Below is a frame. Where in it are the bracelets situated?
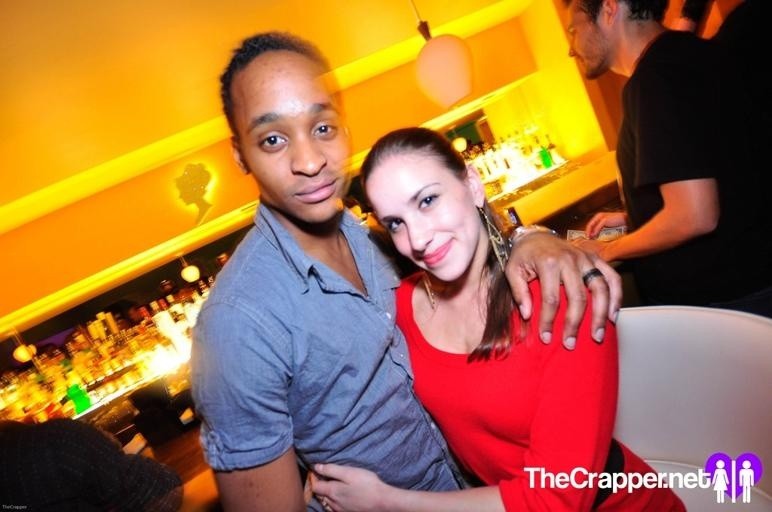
[507,225,556,249]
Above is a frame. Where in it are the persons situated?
[189,32,624,511]
[305,126,686,511]
[567,0,725,317]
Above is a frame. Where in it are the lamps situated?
[410,0,474,110]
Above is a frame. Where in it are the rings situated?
[581,267,605,288]
[318,496,325,504]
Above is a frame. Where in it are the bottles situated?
[0,251,228,422]
[468,129,563,175]
